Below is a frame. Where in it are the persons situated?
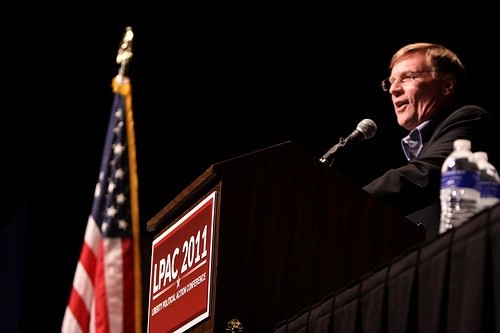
[362,42,500,241]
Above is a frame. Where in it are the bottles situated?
[474,152,500,213]
[440,139,480,234]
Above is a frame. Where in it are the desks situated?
[270,200,500,333]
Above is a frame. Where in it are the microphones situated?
[320,119,378,164]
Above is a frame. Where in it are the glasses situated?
[381,70,431,91]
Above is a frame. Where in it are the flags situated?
[61,75,143,333]
